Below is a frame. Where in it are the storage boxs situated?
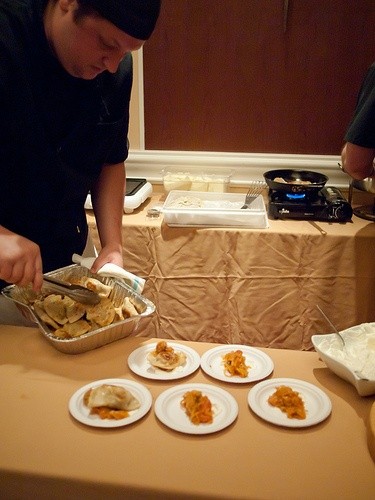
[160,190,270,229]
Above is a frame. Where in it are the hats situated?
[87,0,161,41]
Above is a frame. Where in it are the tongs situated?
[29,272,99,305]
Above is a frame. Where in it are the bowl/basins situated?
[310,322,375,396]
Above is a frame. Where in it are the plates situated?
[127,343,201,380]
[154,383,239,434]
[248,378,332,427]
[69,378,153,428]
[200,345,275,383]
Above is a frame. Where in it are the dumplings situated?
[88,385,140,410]
[32,275,141,340]
[147,351,187,370]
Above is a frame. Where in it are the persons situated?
[342,60,375,179]
[0,0,161,330]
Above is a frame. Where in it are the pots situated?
[262,169,329,194]
[338,162,375,195]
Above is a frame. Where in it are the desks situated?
[82,184,375,352]
[0,323,375,500]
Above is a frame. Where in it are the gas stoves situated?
[267,186,354,223]
